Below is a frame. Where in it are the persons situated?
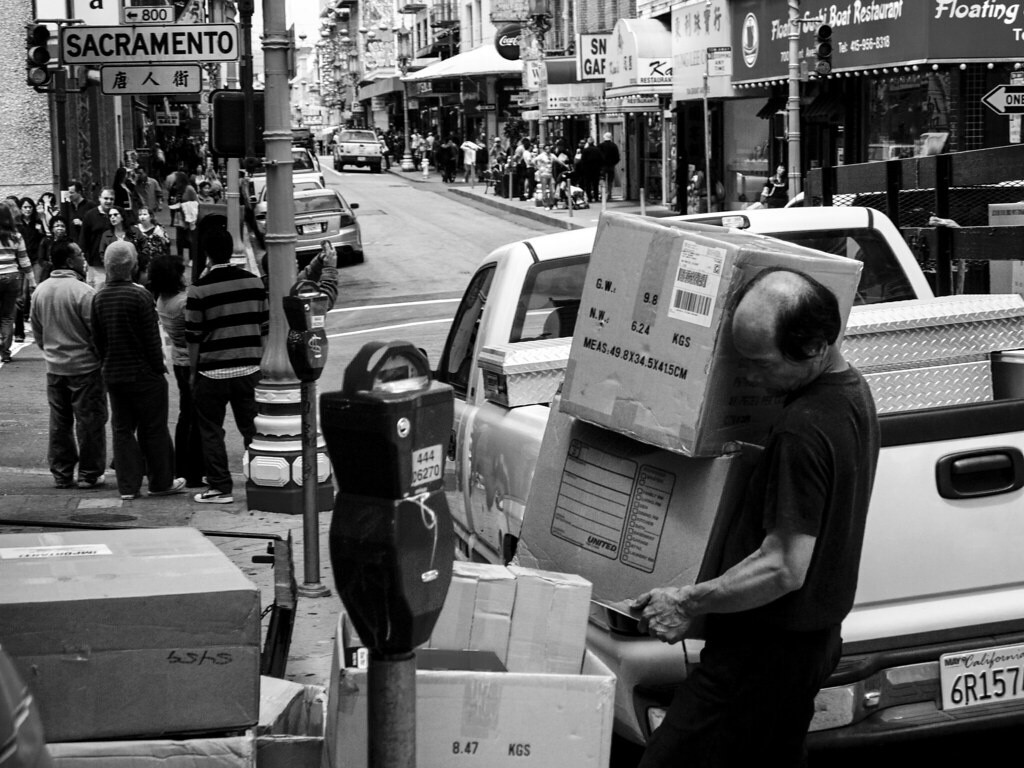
[760,163,788,207]
[627,268,881,768]
[89,241,187,500]
[149,255,208,487]
[30,237,119,490]
[262,243,338,312]
[376,123,488,183]
[505,132,621,210]
[185,230,269,503]
[0,128,222,362]
[490,137,505,159]
[318,131,339,155]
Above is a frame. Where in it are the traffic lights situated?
[812,25,832,75]
[25,24,51,86]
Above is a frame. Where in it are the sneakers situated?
[122,491,141,499]
[193,487,234,503]
[78,475,105,488]
[148,477,186,495]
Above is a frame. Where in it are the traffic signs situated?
[982,86,1024,113]
[473,103,496,113]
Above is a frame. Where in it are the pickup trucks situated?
[332,128,382,172]
[415,205,1024,746]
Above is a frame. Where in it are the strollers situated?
[556,170,589,210]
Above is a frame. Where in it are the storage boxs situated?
[843,295,1024,415]
[477,337,574,407]
[990,203,1023,296]
[510,384,761,625]
[320,560,617,768]
[0,526,328,768]
[559,209,867,457]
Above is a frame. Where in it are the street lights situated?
[527,0,554,206]
[396,18,416,171]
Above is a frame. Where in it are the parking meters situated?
[318,335,455,759]
[283,276,332,600]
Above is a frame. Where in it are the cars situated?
[238,128,364,267]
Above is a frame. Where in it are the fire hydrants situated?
[420,158,429,178]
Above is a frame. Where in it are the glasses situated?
[109,213,120,217]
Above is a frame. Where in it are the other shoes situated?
[3,357,11,362]
[14,334,24,342]
